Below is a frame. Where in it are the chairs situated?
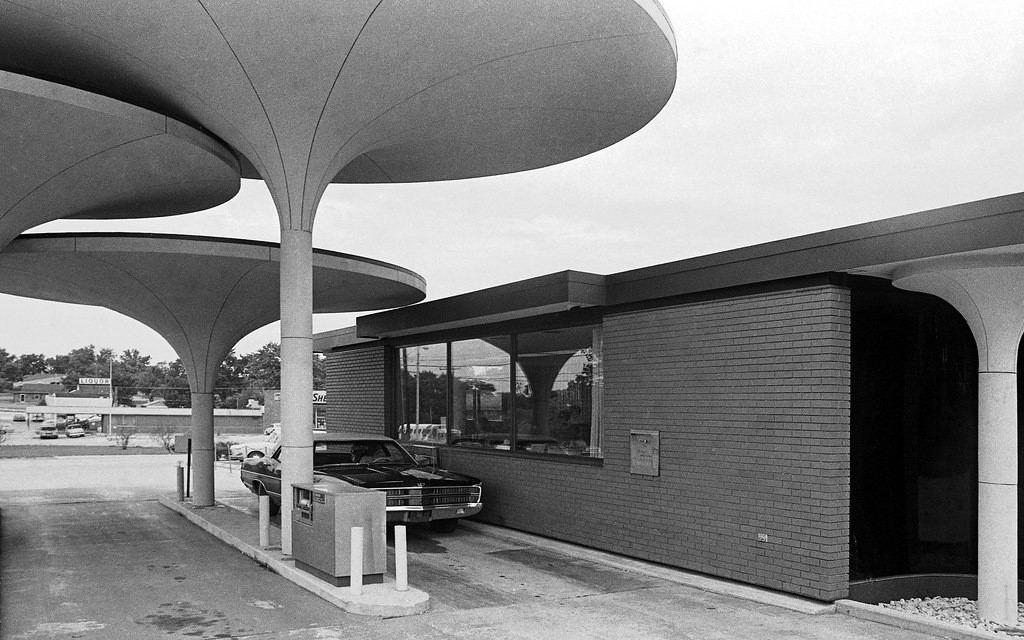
[351,449,376,463]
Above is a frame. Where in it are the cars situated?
[241,432,483,533]
[398,424,591,453]
[40,426,59,439]
[232,431,328,463]
[14,413,26,421]
[65,423,86,438]
[32,414,44,422]
[264,423,281,435]
[42,416,101,433]
